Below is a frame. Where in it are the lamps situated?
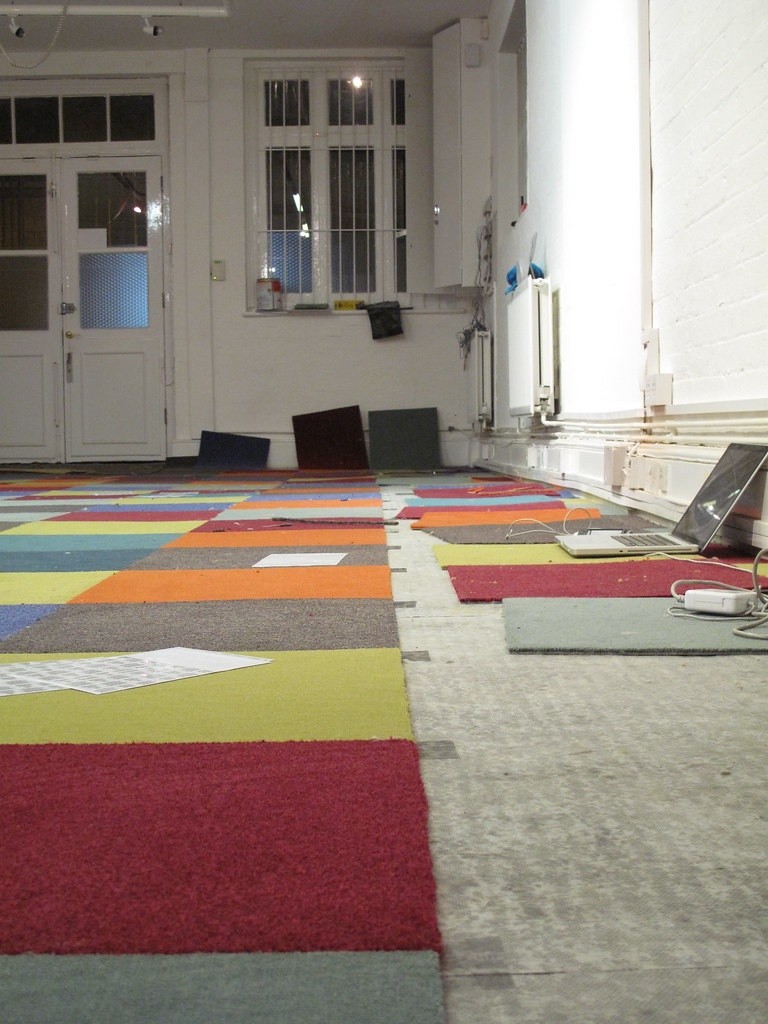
[6,14,26,38]
[142,14,163,36]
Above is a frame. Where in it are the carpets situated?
[0,470,447,1023]
[397,476,768,654]
[290,405,369,471]
[367,407,443,473]
[190,430,271,471]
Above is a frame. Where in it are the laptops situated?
[554,442,768,558]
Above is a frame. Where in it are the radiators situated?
[507,275,555,418]
[467,328,492,424]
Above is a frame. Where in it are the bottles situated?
[257,278,282,311]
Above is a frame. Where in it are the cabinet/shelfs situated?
[404,18,489,298]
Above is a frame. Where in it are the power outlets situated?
[645,371,674,405]
